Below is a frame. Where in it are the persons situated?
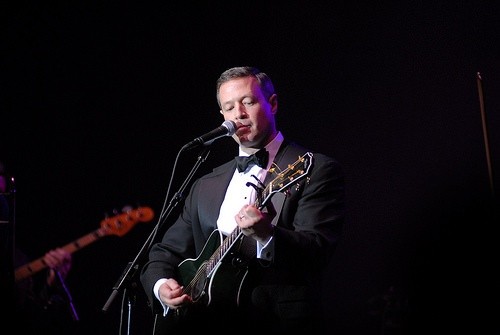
[140,65,342,335]
[0,176,70,335]
[398,186,500,335]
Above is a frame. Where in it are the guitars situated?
[13,205,155,281]
[153,151,315,335]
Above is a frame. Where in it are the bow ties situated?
[235,149,269,173]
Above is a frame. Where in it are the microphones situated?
[182,119,237,150]
